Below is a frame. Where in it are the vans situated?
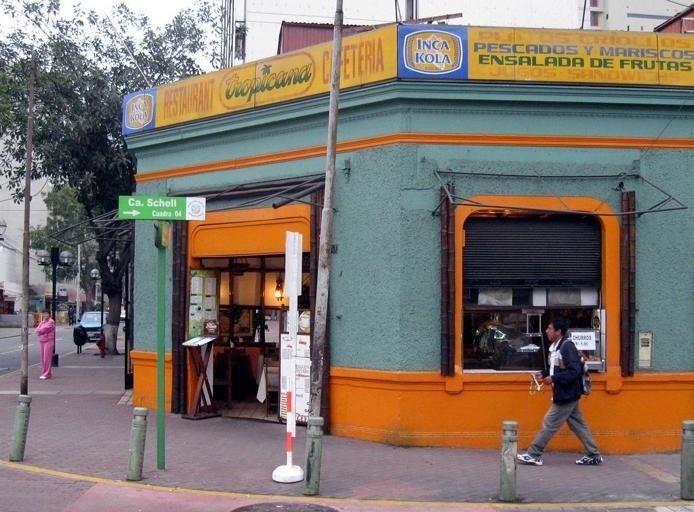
[77,312,108,343]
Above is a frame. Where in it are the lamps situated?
[274,272,284,300]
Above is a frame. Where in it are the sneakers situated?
[575,456,604,467]
[40,376,50,380]
[517,452,544,467]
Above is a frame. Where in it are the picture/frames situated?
[219,308,255,337]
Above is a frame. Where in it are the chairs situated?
[256,353,280,418]
[211,347,232,408]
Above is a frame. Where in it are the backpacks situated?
[559,339,592,396]
[474,325,498,356]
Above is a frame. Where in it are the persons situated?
[35,309,54,380]
[515,316,605,465]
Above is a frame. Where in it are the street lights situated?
[0,216,8,240]
[36,247,73,353]
[90,268,104,358]
